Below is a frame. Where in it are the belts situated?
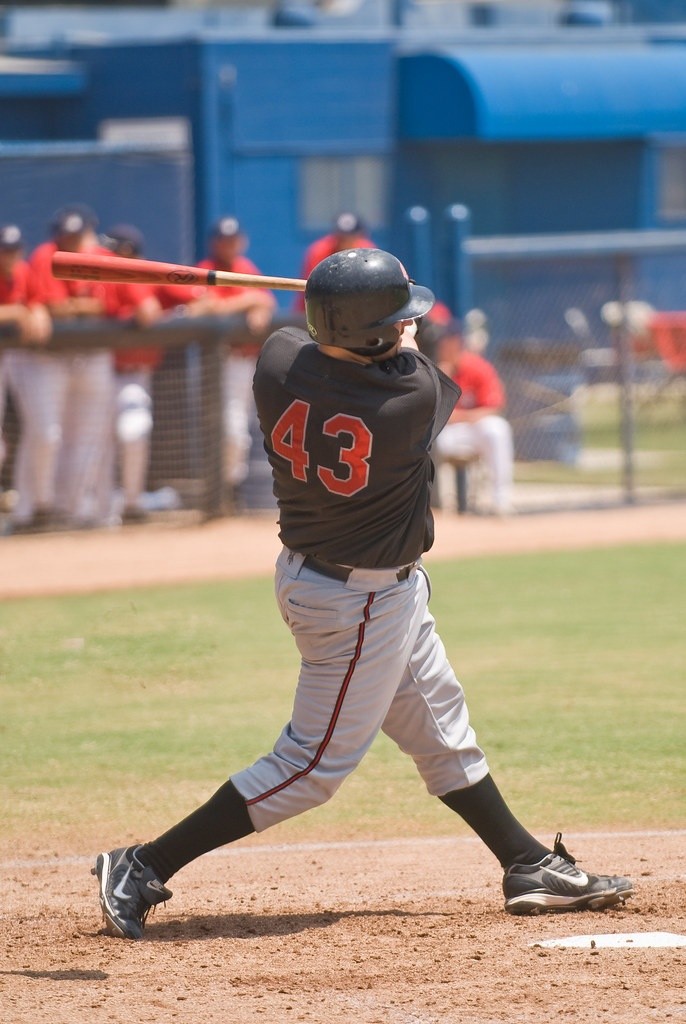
[302,556,411,584]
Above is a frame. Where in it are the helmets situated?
[212,217,240,237]
[0,225,27,248]
[52,206,92,242]
[333,210,364,232]
[101,223,145,250]
[305,247,435,356]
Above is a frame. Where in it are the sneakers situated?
[503,832,636,915]
[92,844,173,940]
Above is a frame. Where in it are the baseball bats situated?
[50,249,309,292]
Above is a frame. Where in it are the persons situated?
[0,203,276,529]
[89,246,634,939]
[612,309,685,392]
[291,212,516,517]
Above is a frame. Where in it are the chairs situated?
[637,309,686,412]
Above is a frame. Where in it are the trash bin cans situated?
[497,339,586,461]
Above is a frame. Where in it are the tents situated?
[399,45,686,146]
